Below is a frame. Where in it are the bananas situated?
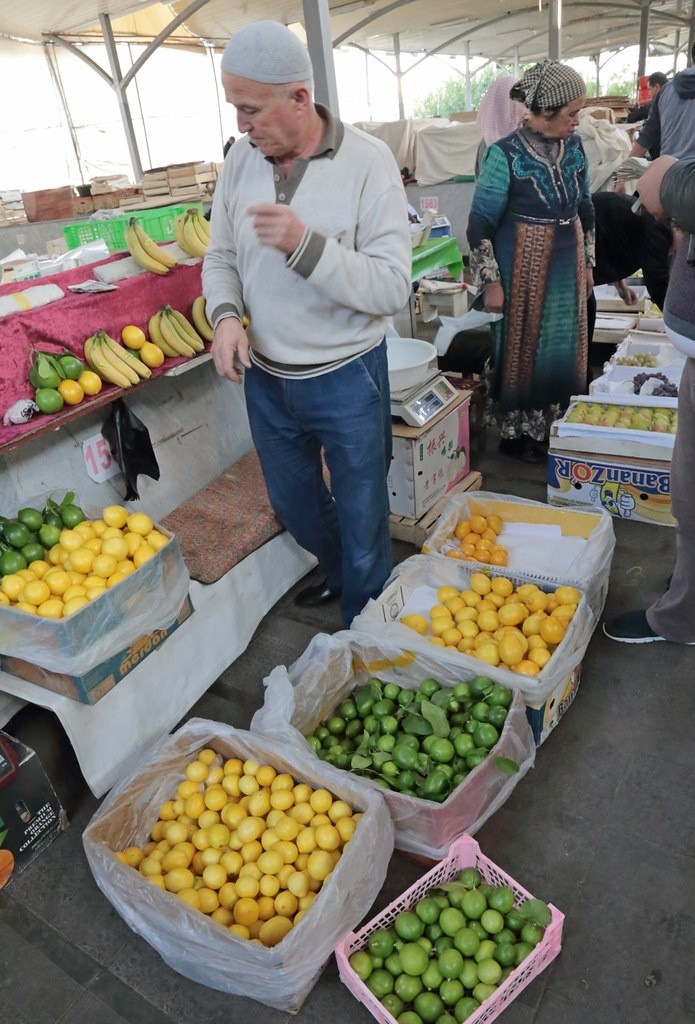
[84,330,152,389]
[148,296,249,357]
[175,208,209,257]
[124,217,178,275]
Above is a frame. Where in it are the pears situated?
[565,403,678,434]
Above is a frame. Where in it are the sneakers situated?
[603,609,695,646]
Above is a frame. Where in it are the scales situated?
[391,369,459,428]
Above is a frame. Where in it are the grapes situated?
[617,351,678,397]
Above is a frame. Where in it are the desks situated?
[412,235,465,339]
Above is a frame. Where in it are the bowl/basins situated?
[386,337,437,392]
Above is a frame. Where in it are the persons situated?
[224,136,235,159]
[602,42,695,644]
[201,20,412,631]
[465,59,595,466]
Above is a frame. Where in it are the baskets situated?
[333,834,566,1024]
[63,201,203,252]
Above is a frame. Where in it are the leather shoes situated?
[498,437,547,465]
[294,578,340,607]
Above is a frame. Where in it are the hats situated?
[221,21,314,84]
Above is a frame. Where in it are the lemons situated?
[0,505,170,618]
[350,868,544,1024]
[114,748,362,948]
[123,325,164,367]
[400,572,580,675]
[29,356,102,413]
[306,676,511,804]
[445,514,508,567]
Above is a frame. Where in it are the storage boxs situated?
[61,207,186,252]
[548,305,687,528]
[333,834,565,1024]
[420,489,617,630]
[1,244,109,285]
[351,551,591,744]
[0,510,195,701]
[387,397,472,520]
[73,195,94,216]
[22,186,74,222]
[93,187,136,212]
[585,108,630,123]
[250,628,537,866]
[425,289,470,320]
[82,715,394,1018]
